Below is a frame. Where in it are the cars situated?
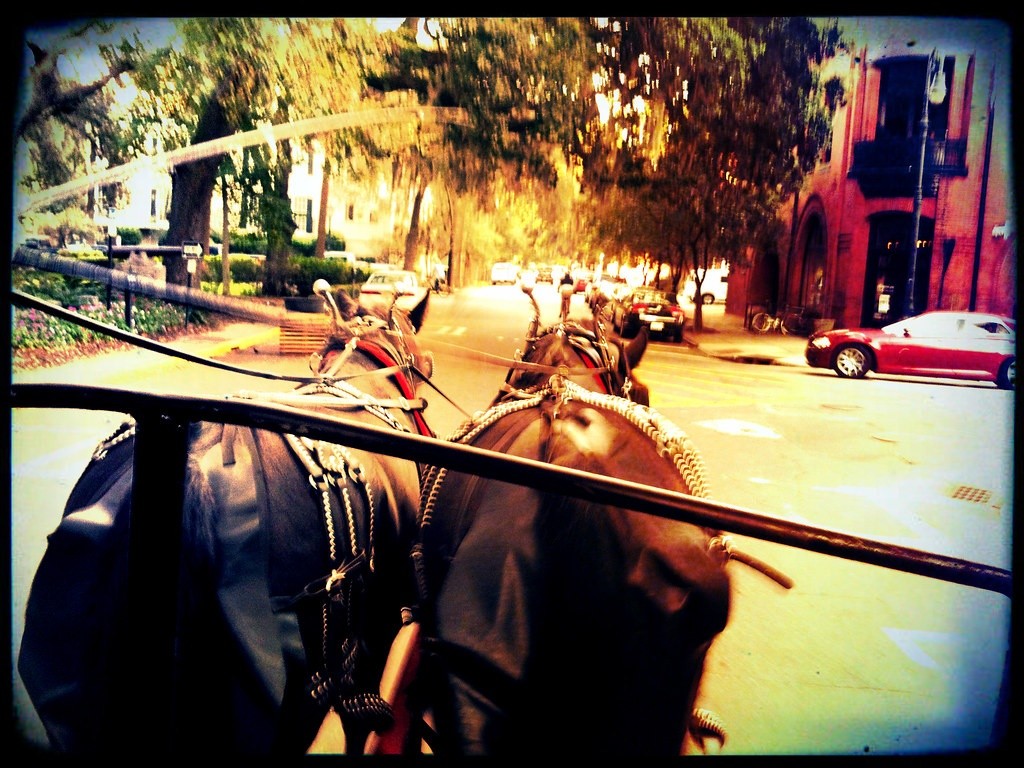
[803,309,1016,390]
[491,262,517,285]
[536,266,634,319]
[610,291,686,344]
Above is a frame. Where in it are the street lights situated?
[907,46,948,314]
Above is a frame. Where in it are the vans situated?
[684,267,730,304]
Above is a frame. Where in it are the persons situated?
[557,274,574,317]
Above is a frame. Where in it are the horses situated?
[63,285,435,758]
[419,321,734,763]
